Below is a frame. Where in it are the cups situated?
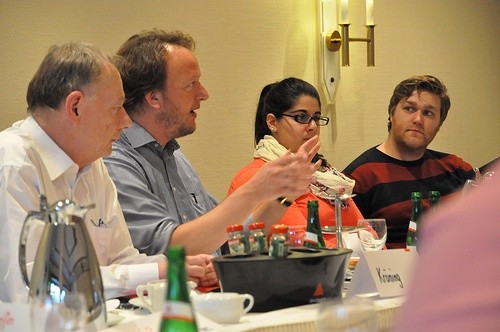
[193,292,254,324]
[357,218,387,250]
[136,279,198,314]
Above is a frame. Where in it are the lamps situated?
[316,0,375,107]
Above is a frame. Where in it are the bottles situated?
[303,200,326,248]
[429,191,440,206]
[226,224,249,256]
[405,191,425,250]
[247,222,269,255]
[158,245,199,332]
[267,224,289,258]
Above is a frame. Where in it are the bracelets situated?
[276,198,293,207]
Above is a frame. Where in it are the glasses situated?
[281,113,330,127]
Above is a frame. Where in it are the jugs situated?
[18,194,108,332]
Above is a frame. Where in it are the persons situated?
[102,30,322,258]
[340,75,476,249]
[0,44,219,304]
[224,78,387,251]
